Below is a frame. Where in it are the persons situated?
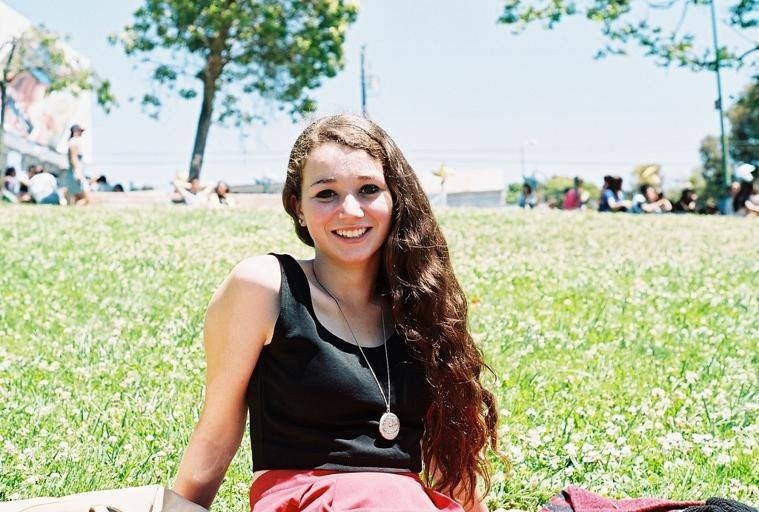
[1,120,231,212]
[166,113,511,512]
[516,160,759,221]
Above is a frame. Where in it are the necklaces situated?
[307,257,400,440]
